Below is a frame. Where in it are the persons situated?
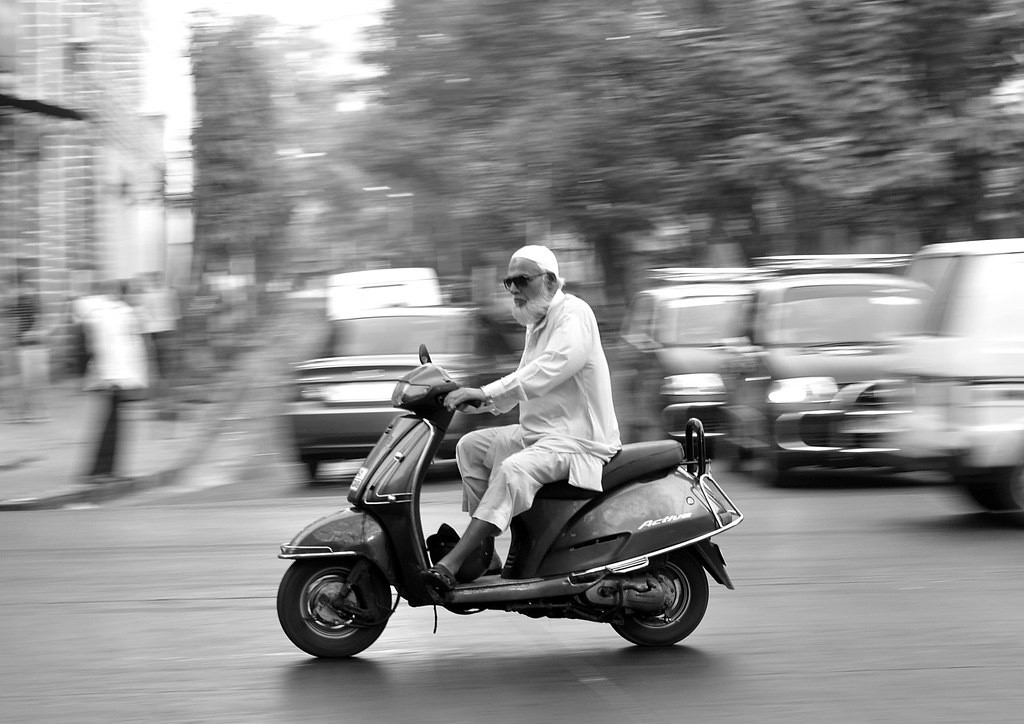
[422,243,624,592]
[74,277,149,480]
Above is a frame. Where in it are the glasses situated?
[503,272,556,291]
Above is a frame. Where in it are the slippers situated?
[420,564,456,592]
[483,569,502,576]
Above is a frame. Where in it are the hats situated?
[511,245,560,280]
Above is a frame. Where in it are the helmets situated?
[427,522,495,584]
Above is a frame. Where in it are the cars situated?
[613,239,1024,512]
[279,266,522,482]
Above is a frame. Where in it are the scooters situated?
[277,345,746,660]
